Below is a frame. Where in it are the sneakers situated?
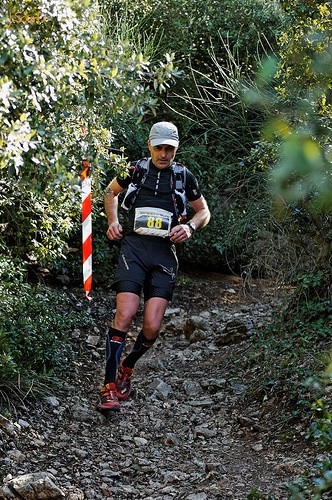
[117,361,134,399]
[96,383,119,409]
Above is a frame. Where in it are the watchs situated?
[187,221,196,234]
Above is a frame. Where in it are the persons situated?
[98,121,212,410]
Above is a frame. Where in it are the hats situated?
[149,121,179,148]
